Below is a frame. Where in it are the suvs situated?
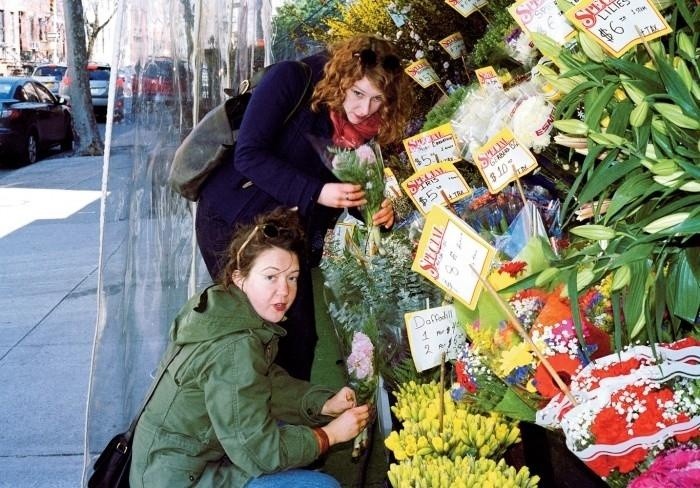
[130,55,188,107]
[59,62,126,122]
[31,64,68,97]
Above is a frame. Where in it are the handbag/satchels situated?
[168,81,256,202]
[87,433,135,487]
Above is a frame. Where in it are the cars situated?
[0,78,73,164]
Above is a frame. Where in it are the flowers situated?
[259,0,700,488]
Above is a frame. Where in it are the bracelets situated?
[315,425,331,453]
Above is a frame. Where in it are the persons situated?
[194,37,413,383]
[128,208,377,487]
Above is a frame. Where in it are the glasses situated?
[353,52,404,73]
[236,224,304,272]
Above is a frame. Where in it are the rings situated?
[345,191,350,200]
[360,422,364,432]
